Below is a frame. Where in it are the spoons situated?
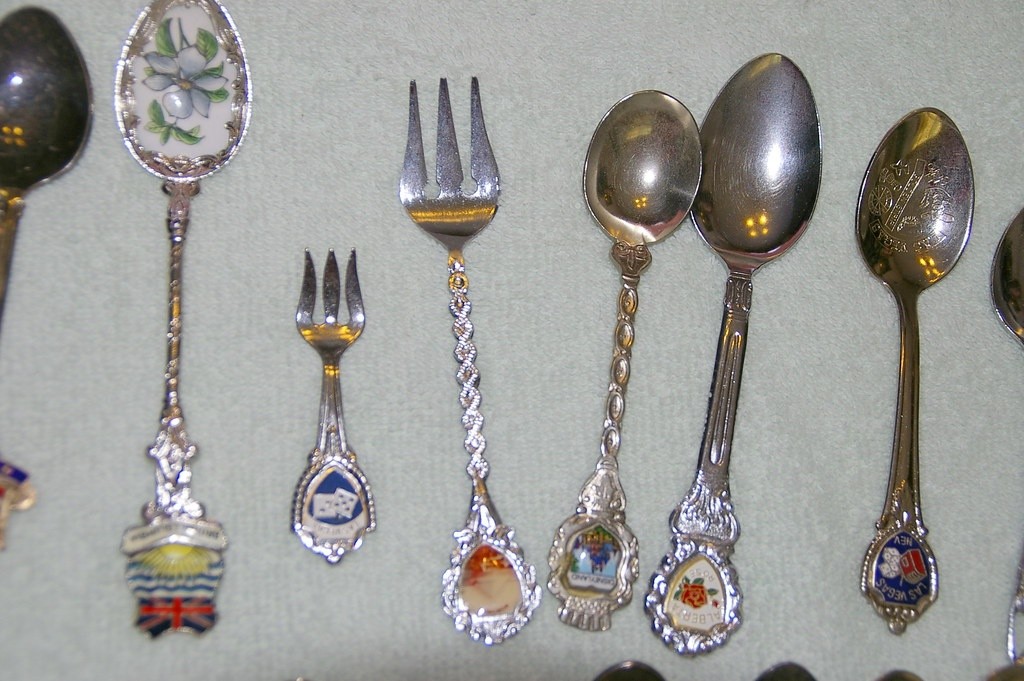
[0,8,96,545]
[875,670,924,681]
[755,662,817,681]
[594,660,666,681]
[853,107,975,636]
[646,51,821,656]
[549,90,702,630]
[989,209,1024,667]
[115,0,253,640]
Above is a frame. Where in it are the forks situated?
[398,76,541,646]
[287,246,376,565]
